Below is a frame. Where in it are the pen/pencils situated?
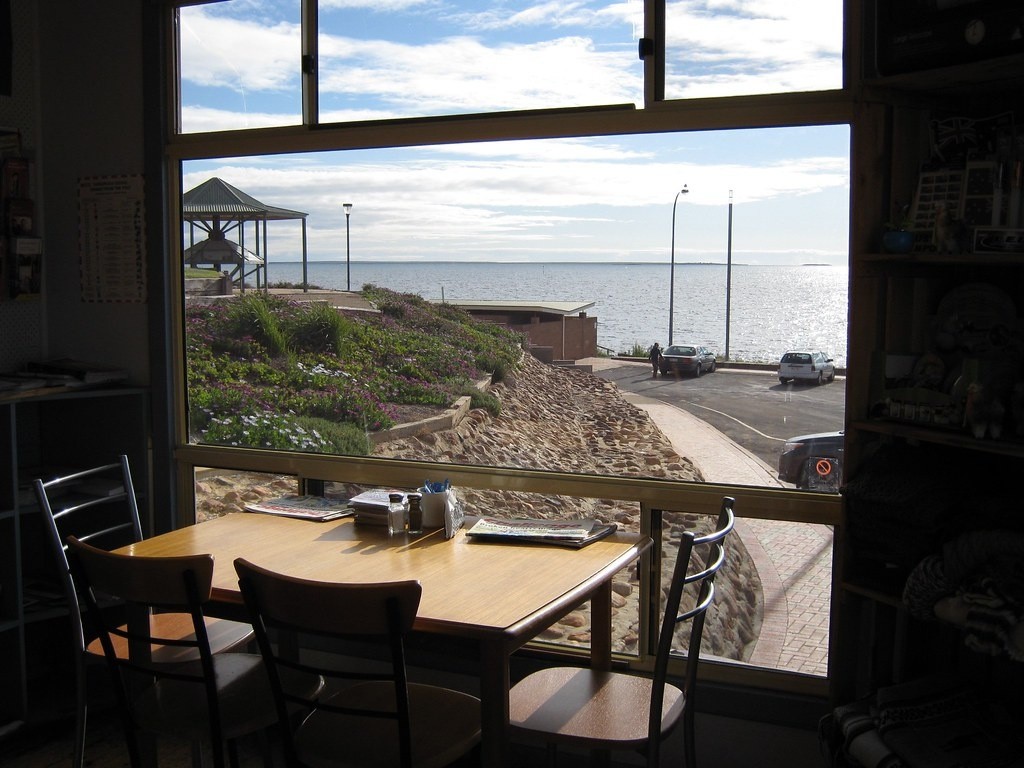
[423,478,450,493]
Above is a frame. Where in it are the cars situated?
[778,428,845,492]
[659,344,717,378]
[778,349,835,386]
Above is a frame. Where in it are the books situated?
[347,489,422,525]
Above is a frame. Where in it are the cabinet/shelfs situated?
[840,248,1024,662]
[0,387,158,748]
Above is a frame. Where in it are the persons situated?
[649,343,664,377]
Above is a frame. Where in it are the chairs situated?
[38,453,736,768]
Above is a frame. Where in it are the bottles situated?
[387,494,406,537]
[407,493,423,535]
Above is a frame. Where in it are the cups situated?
[417,486,445,527]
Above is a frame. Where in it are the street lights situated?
[723,188,733,363]
[342,202,353,292]
[668,184,689,346]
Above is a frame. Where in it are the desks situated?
[106,507,649,768]
